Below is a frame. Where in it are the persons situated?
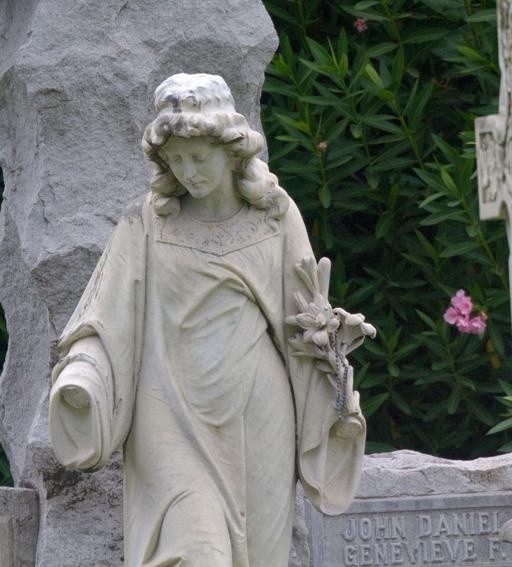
[49,72,376,567]
